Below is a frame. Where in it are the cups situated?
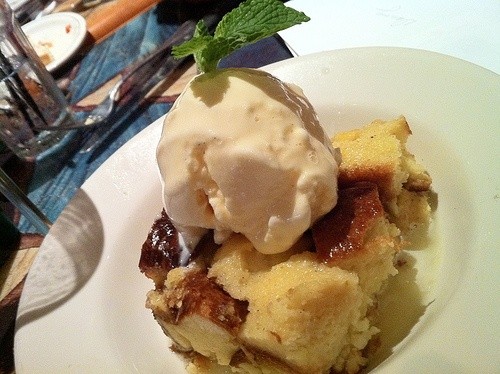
[0,0,76,161]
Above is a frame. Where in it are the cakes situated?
[138,69,434,374]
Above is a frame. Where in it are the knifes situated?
[79,15,219,153]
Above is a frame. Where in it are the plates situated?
[0,11,87,77]
[13,46,500,374]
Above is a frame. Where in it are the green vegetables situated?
[171,0,311,74]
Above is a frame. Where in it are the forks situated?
[31,20,196,131]
